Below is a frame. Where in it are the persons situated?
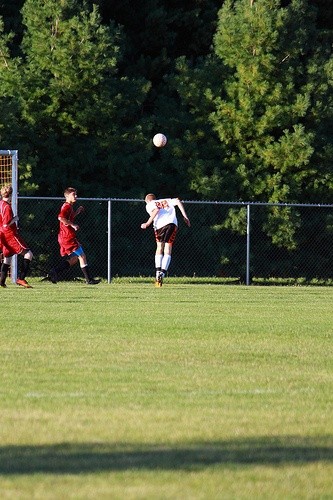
[49,187,101,285]
[140,194,190,287]
[0,186,33,288]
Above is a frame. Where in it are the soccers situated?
[153,133,167,147]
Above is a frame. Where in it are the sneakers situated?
[155,274,163,287]
[47,270,57,284]
[16,277,33,288]
[0,282,7,288]
[85,278,100,284]
